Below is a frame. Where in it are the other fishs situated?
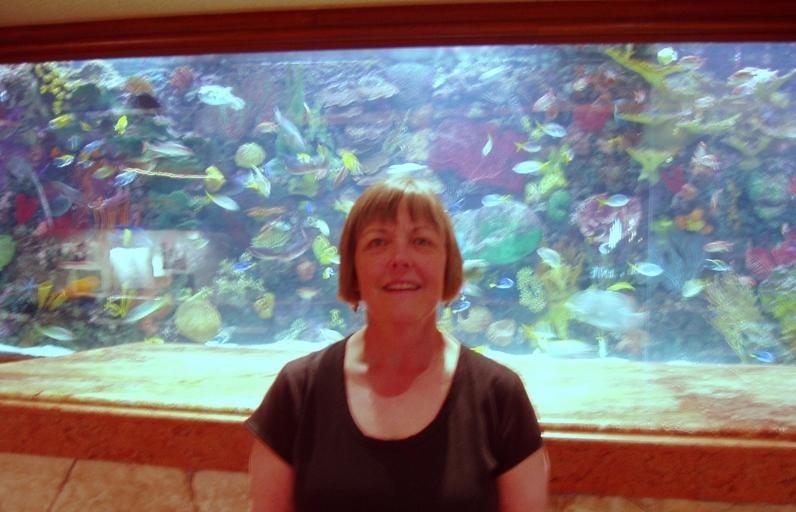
[1,42,796,364]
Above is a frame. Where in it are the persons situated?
[243,175,551,512]
